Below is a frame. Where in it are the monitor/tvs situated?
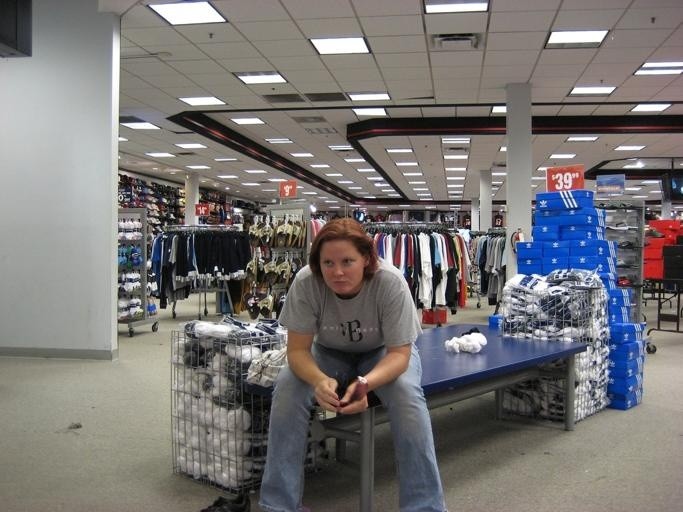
[354,211,365,223]
[0,0,33,58]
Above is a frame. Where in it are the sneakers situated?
[195,489,252,511]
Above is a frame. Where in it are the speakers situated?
[663,173,683,201]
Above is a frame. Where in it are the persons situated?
[257,217,449,511]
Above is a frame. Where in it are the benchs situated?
[315,324,587,512]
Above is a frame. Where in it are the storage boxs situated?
[489,188,683,409]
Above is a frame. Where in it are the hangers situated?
[471,229,506,242]
[361,220,460,239]
[155,224,247,242]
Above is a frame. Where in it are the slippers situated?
[244,221,306,320]
